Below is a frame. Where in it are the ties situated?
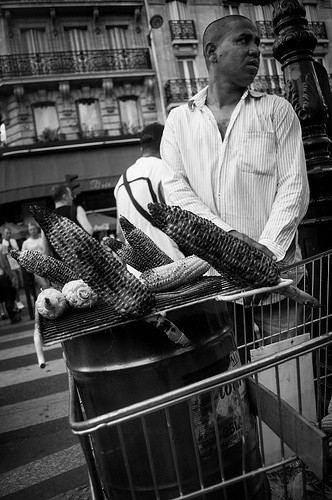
[7,241,13,253]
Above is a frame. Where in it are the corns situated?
[8,198,323,348]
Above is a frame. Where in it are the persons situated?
[160,15,312,347]
[115,122,187,273]
[45,184,95,289]
[3,227,30,310]
[24,223,55,293]
[0,233,24,324]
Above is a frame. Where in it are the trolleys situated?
[66,248,331,500]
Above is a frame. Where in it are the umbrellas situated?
[87,211,118,232]
